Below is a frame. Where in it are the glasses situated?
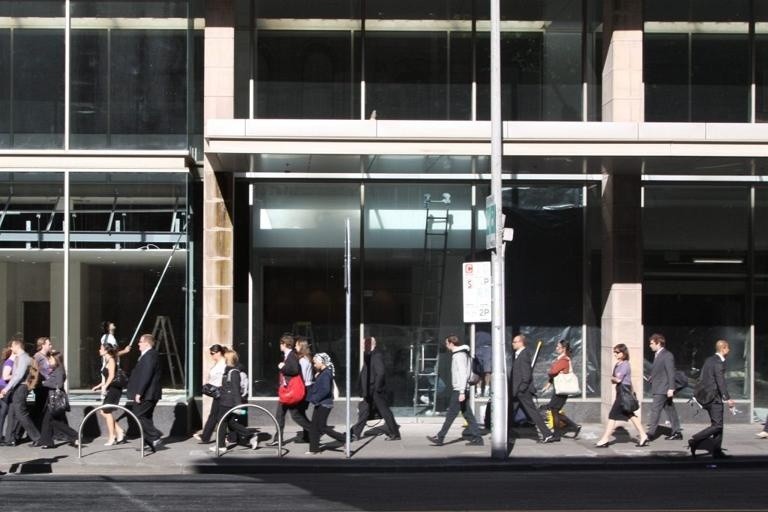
[614,351,622,354]
[210,352,216,354]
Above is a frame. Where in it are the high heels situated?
[593,442,609,448]
[103,429,126,446]
[635,437,650,447]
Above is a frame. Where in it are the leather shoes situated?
[573,425,581,438]
[265,440,284,447]
[344,432,359,441]
[0,441,17,446]
[665,432,682,440]
[136,438,165,453]
[27,442,51,448]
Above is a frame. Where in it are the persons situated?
[192,343,258,452]
[643,334,684,442]
[265,334,313,446]
[425,333,483,447]
[91,321,131,446]
[127,333,166,452]
[306,352,347,453]
[1,336,79,449]
[543,338,583,442]
[688,338,737,461]
[351,332,398,443]
[756,414,768,438]
[595,342,650,448]
[507,335,553,444]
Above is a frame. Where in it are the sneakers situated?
[192,433,202,441]
[465,440,484,445]
[249,432,259,450]
[688,439,697,457]
[209,445,228,453]
[427,435,443,445]
[755,430,767,438]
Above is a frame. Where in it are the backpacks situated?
[228,368,248,398]
[452,350,484,385]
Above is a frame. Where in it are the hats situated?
[46,349,60,356]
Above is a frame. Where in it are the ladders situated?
[151,315,185,389]
[292,321,317,357]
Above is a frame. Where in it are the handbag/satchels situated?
[618,382,639,412]
[112,368,128,389]
[278,375,306,405]
[691,383,716,409]
[48,388,70,415]
[202,384,222,400]
[674,371,687,389]
[553,373,582,396]
